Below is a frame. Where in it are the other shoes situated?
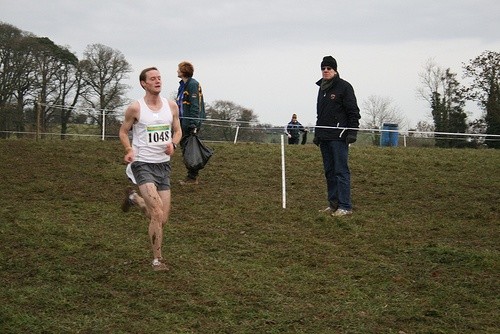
[332,209,353,217]
[151,258,169,272]
[318,206,336,213]
[121,186,137,212]
[178,174,199,186]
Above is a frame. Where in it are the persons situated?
[117,67,182,274]
[175,60,215,187]
[285,113,309,146]
[312,56,362,218]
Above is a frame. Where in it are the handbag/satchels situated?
[180,129,214,171]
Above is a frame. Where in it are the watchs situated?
[170,141,179,152]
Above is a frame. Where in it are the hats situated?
[292,114,297,117]
[321,56,337,71]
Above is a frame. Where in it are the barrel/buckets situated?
[381,123,399,147]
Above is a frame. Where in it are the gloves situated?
[304,129,309,132]
[288,134,292,138]
[313,137,319,145]
[346,131,357,143]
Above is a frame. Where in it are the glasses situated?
[322,67,331,70]
